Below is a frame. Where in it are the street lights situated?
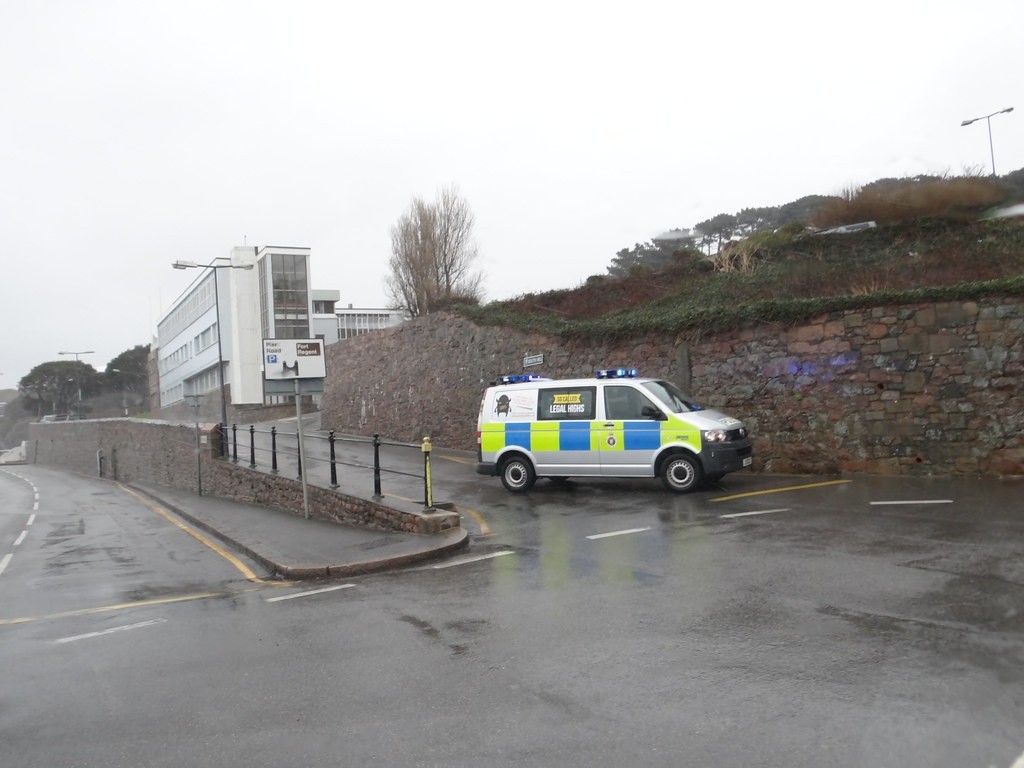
[58,350,95,420]
[959,106,1014,176]
[169,260,254,463]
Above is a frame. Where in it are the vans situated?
[474,368,753,495]
